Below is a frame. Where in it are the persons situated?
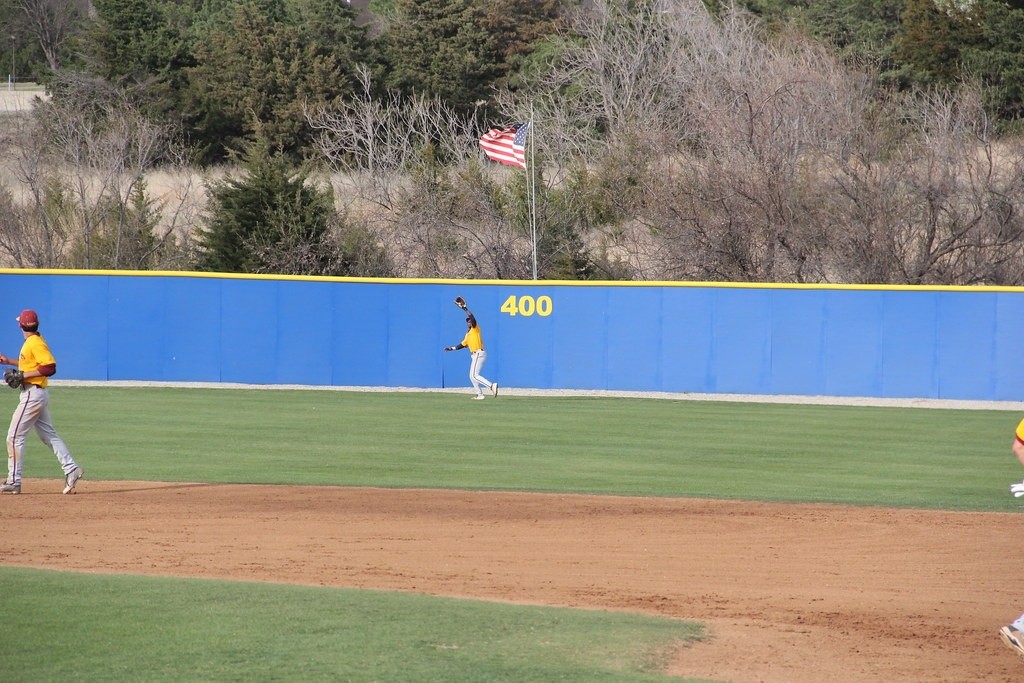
[999,418,1024,656]
[446,303,498,399]
[0,308,84,494]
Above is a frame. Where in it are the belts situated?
[474,349,483,353]
[23,385,41,390]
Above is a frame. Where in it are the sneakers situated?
[0,482,20,495]
[999,624,1024,655]
[491,383,498,397]
[471,395,485,400]
[63,467,84,494]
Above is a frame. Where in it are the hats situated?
[16,310,37,327]
[466,317,470,322]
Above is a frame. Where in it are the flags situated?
[479,122,531,171]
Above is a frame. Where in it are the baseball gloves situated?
[453,296,467,308]
[3,368,24,389]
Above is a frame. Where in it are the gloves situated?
[1010,483,1024,497]
